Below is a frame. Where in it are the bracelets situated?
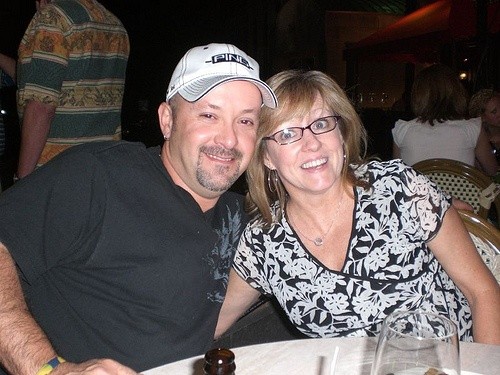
[36,356,66,375]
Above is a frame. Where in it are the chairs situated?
[413,158,500,288]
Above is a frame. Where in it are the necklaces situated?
[287,188,343,245]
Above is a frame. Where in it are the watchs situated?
[13,173,19,183]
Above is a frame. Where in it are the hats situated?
[166,43,278,110]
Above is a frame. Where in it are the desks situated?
[144,335,500,375]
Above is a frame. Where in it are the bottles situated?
[201,348,237,375]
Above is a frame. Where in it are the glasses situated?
[262,115,342,145]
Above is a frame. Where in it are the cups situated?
[350,88,396,108]
[369,311,460,375]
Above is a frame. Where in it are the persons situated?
[0,42,474,375]
[0,0,130,186]
[391,64,499,226]
[214,67,500,346]
